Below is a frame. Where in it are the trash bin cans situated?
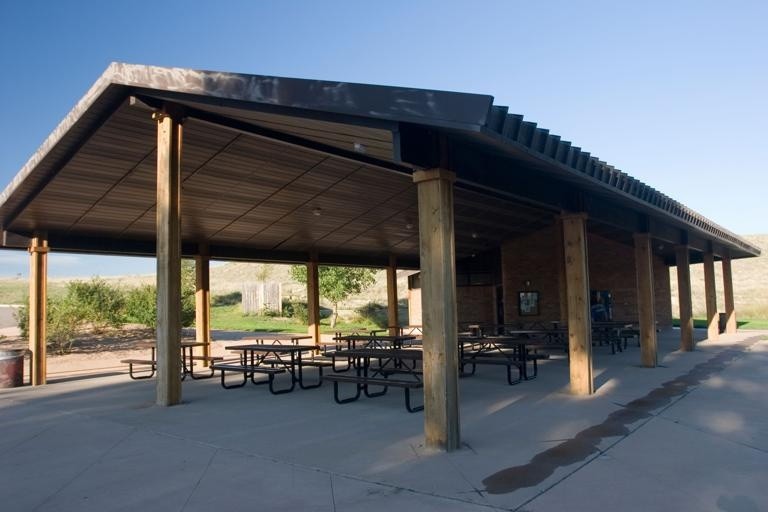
[469,325,479,336]
[0,348,24,388]
[719,313,726,333]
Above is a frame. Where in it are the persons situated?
[590,291,608,322]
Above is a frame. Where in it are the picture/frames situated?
[519,291,539,316]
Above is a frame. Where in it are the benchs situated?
[120,321,661,413]
